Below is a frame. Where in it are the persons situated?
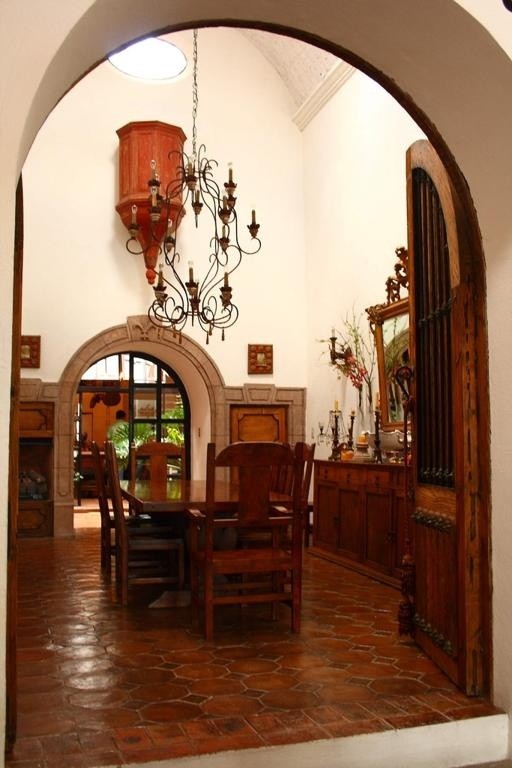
[107,410,130,480]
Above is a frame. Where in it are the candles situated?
[358,431,367,443]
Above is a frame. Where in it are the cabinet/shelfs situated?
[308,458,414,591]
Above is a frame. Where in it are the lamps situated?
[122,29,264,346]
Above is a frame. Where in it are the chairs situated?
[105,441,185,609]
[305,443,316,502]
[129,443,192,519]
[92,441,153,586]
[185,442,304,644]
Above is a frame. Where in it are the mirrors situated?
[365,247,413,432]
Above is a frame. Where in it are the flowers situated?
[381,318,411,422]
[314,300,378,415]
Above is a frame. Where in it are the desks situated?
[119,479,295,603]
